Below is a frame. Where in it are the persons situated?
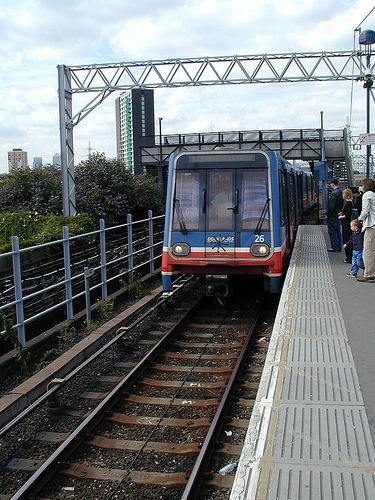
[357,178,375,282]
[342,219,366,278]
[351,189,359,220]
[356,191,364,217]
[212,180,232,219]
[338,188,353,263]
[325,180,343,252]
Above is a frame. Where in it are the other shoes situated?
[357,277,375,283]
[343,258,348,263]
[345,273,356,278]
[327,248,341,253]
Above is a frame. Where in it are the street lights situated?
[357,29,375,180]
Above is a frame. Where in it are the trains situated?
[160,151,321,305]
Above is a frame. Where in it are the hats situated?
[328,178,339,184]
